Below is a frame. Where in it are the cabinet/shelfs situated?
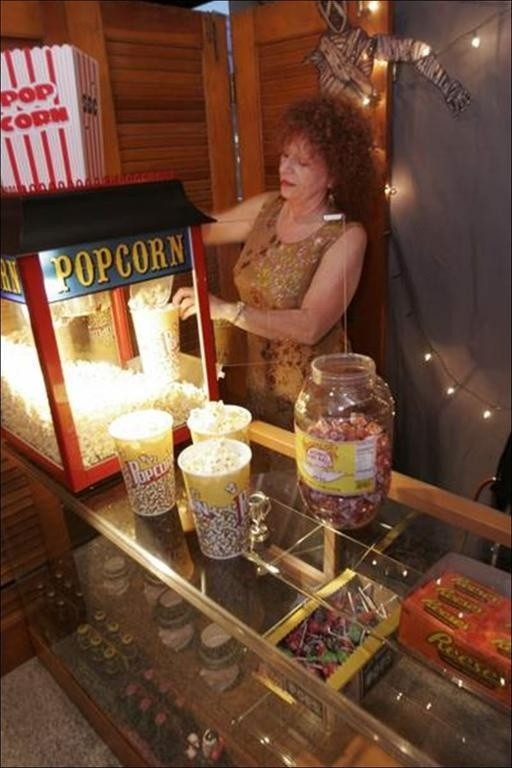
[1,439,512,768]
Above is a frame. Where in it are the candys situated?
[298,413,394,532]
[284,581,398,684]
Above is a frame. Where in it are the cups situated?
[130,303,182,385]
[107,408,178,516]
[187,403,253,448]
[177,436,253,560]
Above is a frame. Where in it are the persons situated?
[172,94,378,435]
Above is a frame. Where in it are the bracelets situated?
[228,297,248,328]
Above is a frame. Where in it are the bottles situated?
[294,351,396,530]
[30,554,241,767]
